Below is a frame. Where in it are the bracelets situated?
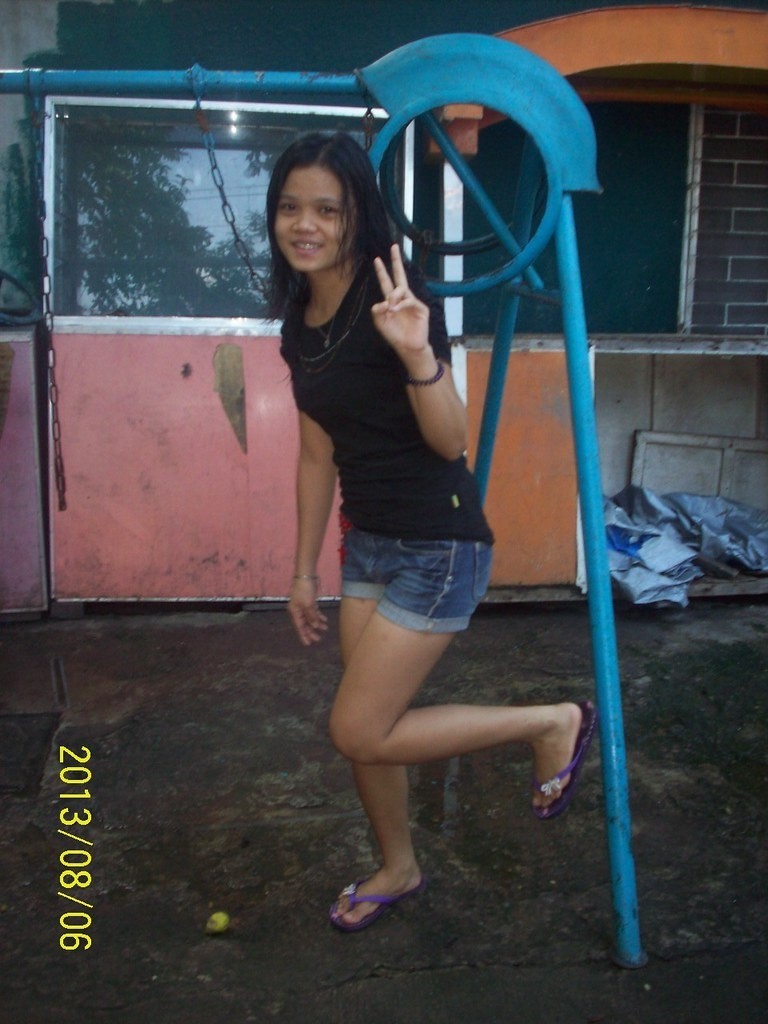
[293,575,319,580]
[407,360,445,386]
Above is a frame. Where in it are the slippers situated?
[328,874,425,931]
[530,696,597,819]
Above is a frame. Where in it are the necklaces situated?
[307,286,348,348]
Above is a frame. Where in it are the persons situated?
[261,132,599,934]
